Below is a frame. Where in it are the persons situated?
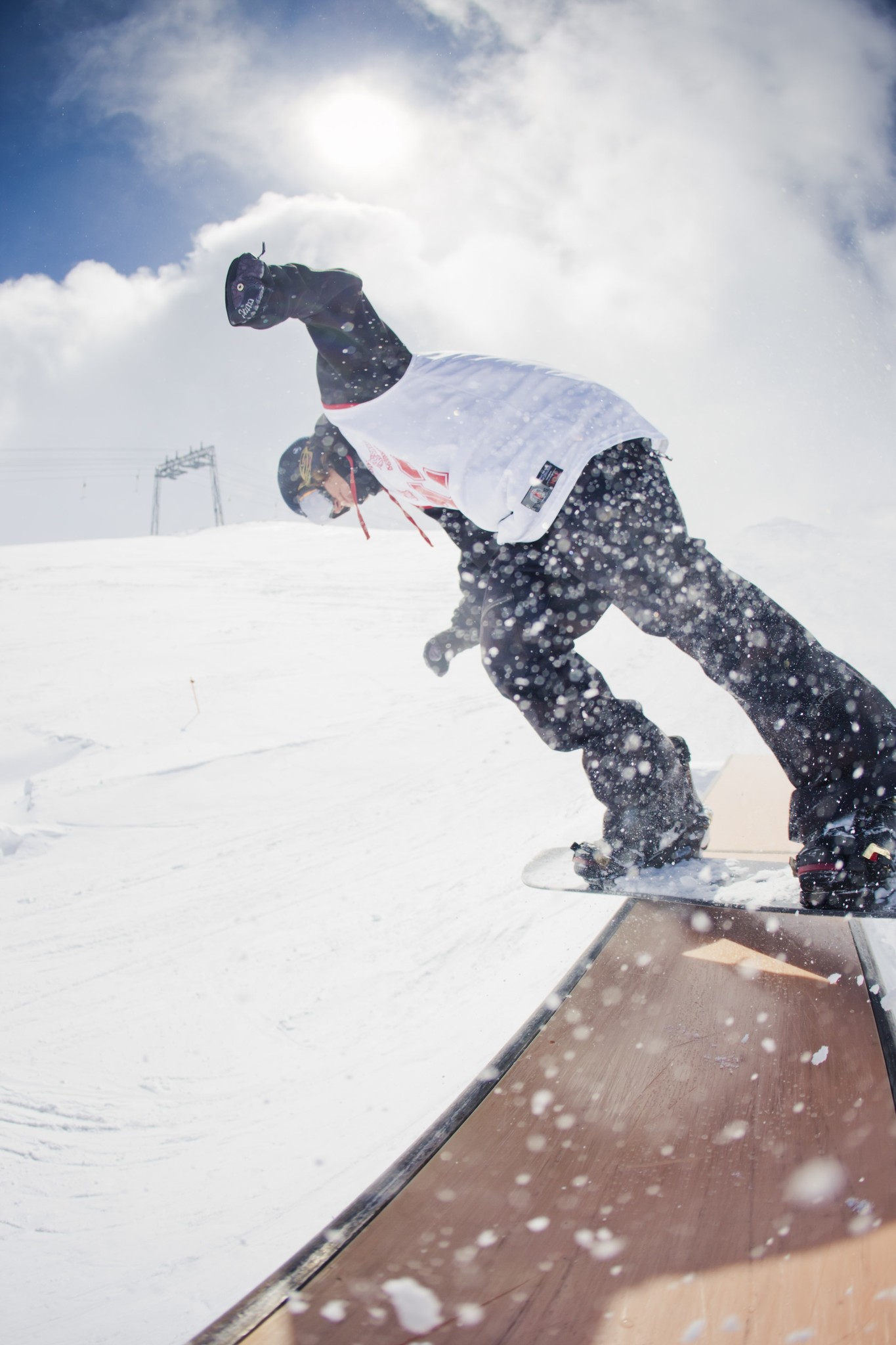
[222,241,896,915]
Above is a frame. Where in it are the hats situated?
[277,436,311,519]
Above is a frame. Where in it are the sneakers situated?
[574,814,711,889]
[792,807,895,909]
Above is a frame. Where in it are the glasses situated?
[298,487,334,524]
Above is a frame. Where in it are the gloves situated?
[226,253,273,327]
[425,625,477,677]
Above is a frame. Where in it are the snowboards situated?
[523,844,896,920]
